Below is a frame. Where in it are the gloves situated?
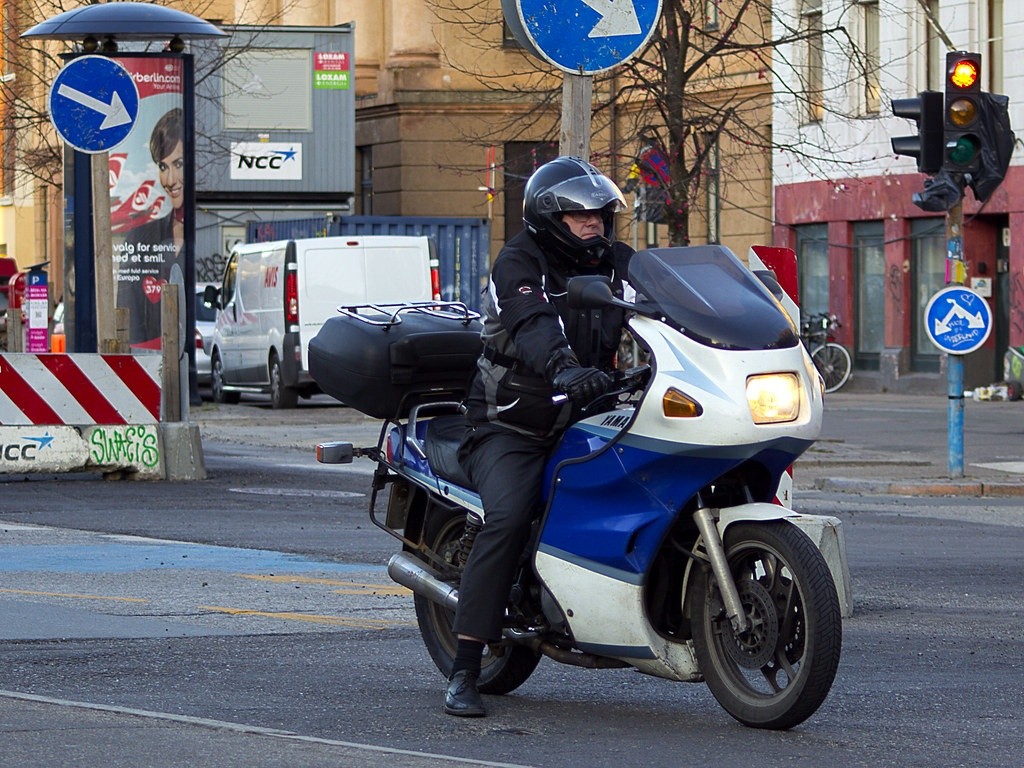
[552,367,614,410]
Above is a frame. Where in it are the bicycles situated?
[799,313,851,393]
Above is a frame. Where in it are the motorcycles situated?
[308,244,842,731]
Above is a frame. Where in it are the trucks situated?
[0,257,19,317]
[245,211,491,323]
[204,235,440,410]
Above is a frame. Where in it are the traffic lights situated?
[981,92,1015,171]
[891,90,943,173]
[943,51,983,173]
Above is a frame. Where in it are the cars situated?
[808,273,885,327]
[194,282,223,383]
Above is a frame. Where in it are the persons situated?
[445,156,635,716]
[115,108,185,347]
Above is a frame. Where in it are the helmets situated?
[523,156,618,270]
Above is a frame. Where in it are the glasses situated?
[564,211,603,224]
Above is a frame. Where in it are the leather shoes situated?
[443,670,487,716]
